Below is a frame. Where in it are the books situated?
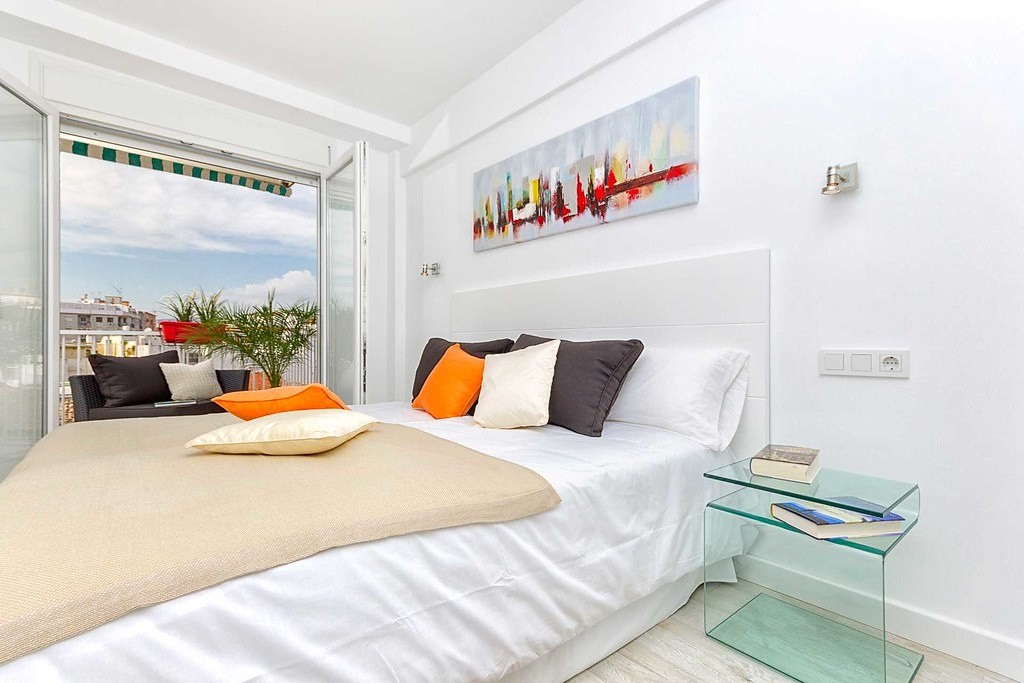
[771,497,905,540]
[750,444,822,484]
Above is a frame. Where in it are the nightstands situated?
[702,456,925,683]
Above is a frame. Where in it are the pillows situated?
[158,357,225,401]
[410,343,485,420]
[210,382,353,421]
[184,408,380,455]
[472,339,561,429]
[508,331,645,438]
[604,347,750,453]
[411,337,515,416]
[88,349,179,408]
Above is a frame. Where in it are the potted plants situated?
[149,287,225,343]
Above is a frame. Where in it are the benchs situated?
[67,369,250,423]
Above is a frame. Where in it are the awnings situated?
[60,131,292,197]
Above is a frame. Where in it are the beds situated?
[0,247,771,683]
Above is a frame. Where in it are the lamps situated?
[820,162,860,197]
[419,262,440,277]
